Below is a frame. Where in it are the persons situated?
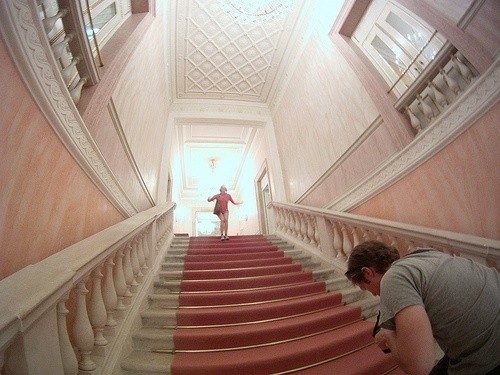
[345,239,500,375]
[207,186,242,240]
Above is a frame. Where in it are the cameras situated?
[373,312,393,352]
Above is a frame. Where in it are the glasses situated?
[344,262,376,281]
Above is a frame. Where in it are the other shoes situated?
[221,235,224,239]
[225,236,229,239]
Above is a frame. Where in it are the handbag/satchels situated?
[214,200,221,214]
